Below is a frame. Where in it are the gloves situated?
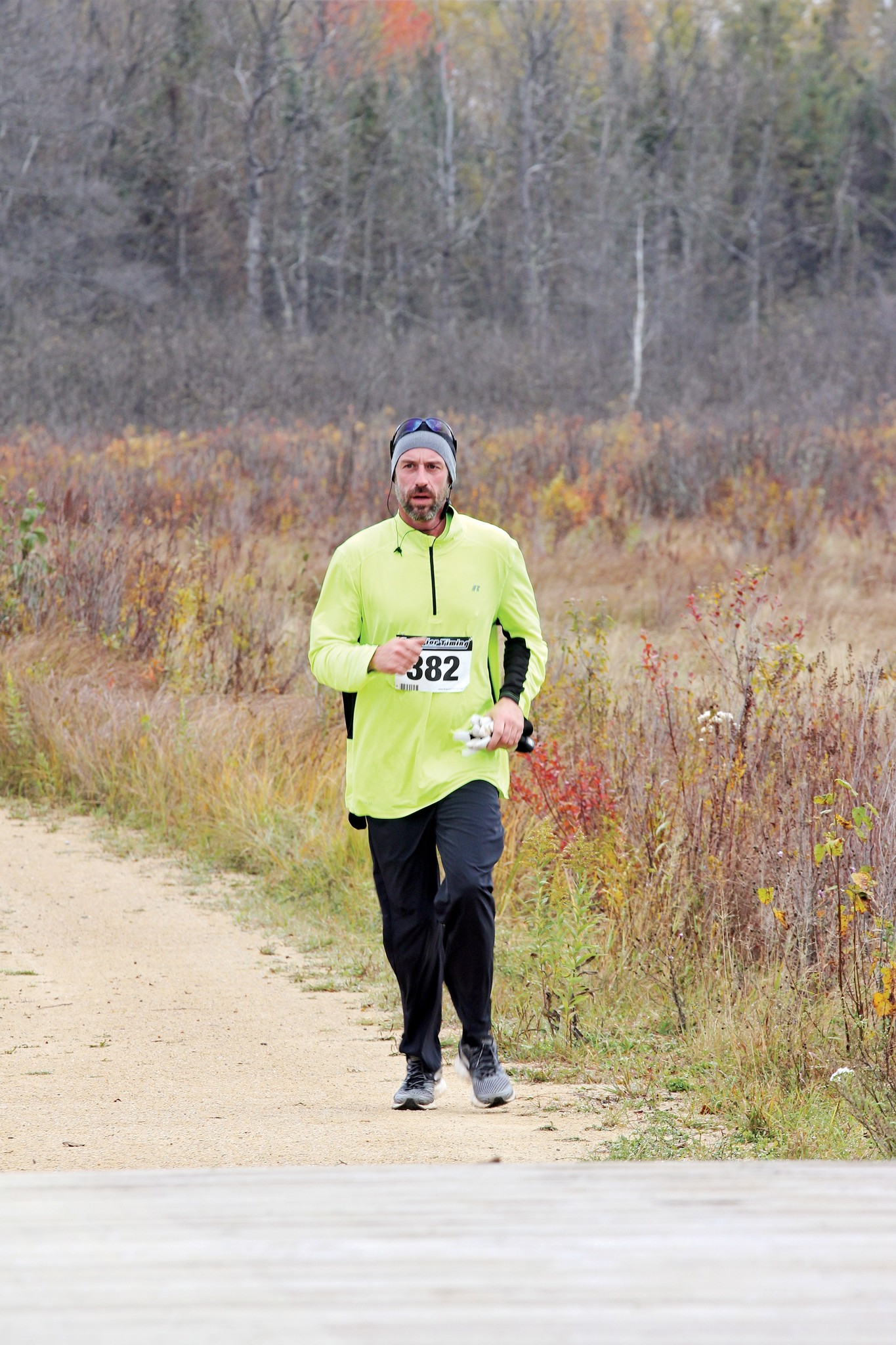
[453,714,535,752]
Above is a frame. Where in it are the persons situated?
[307,418,549,1109]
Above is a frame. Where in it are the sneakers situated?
[455,1033,515,1108]
[391,1054,447,1109]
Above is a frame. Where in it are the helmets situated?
[389,417,457,460]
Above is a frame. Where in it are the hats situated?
[391,422,456,490]
